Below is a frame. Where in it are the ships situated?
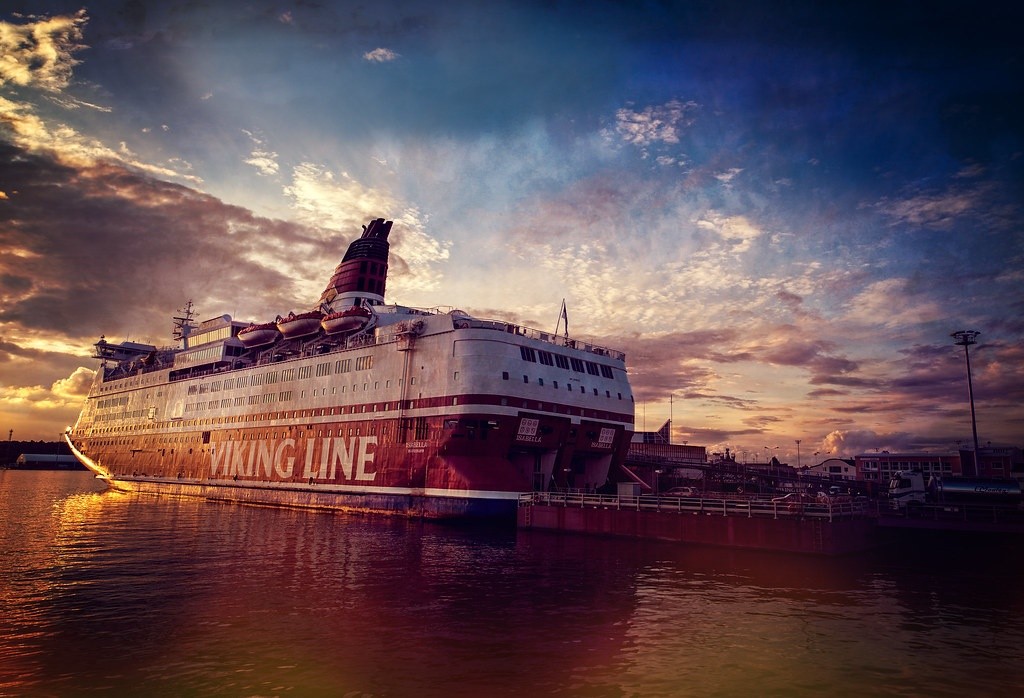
[64,216,636,523]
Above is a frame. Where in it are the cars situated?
[831,492,853,500]
[662,487,700,497]
[772,493,816,504]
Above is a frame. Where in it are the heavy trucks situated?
[887,469,1021,519]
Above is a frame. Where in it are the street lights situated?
[950,329,983,475]
[725,440,831,466]
[655,470,663,513]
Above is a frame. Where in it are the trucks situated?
[829,481,848,495]
